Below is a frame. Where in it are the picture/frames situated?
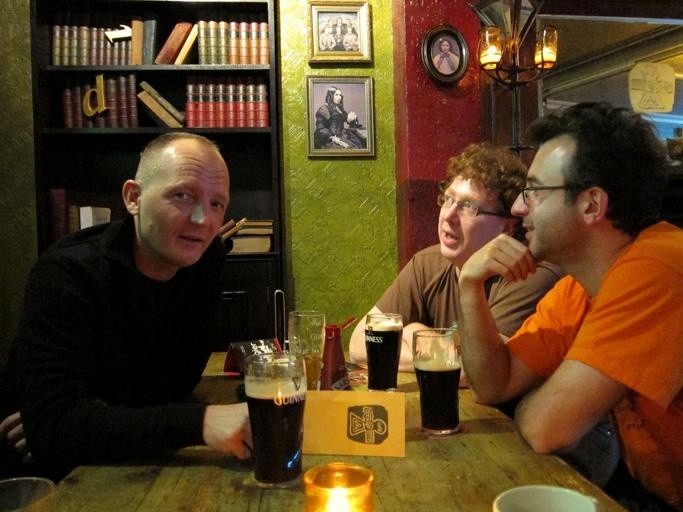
[420,24,470,85]
[306,0,374,66]
[306,75,376,157]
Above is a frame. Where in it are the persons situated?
[457,101,682,512]
[317,16,358,51]
[1,130,256,477]
[313,87,368,150]
[432,40,462,75]
[343,142,618,488]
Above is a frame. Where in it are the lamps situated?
[464,0,559,158]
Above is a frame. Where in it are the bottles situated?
[320,325,351,390]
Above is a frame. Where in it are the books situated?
[64,74,270,128]
[41,20,270,65]
[49,189,111,242]
[218,218,271,254]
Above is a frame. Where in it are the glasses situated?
[520,184,567,203]
[437,194,506,218]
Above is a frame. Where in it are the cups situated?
[364,312,402,392]
[287,309,325,390]
[299,462,380,512]
[0,477,58,512]
[415,329,464,438]
[240,352,308,484]
[487,483,597,512]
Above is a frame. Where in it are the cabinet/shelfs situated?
[30,0,283,352]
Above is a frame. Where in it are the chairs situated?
[0,411,33,464]
